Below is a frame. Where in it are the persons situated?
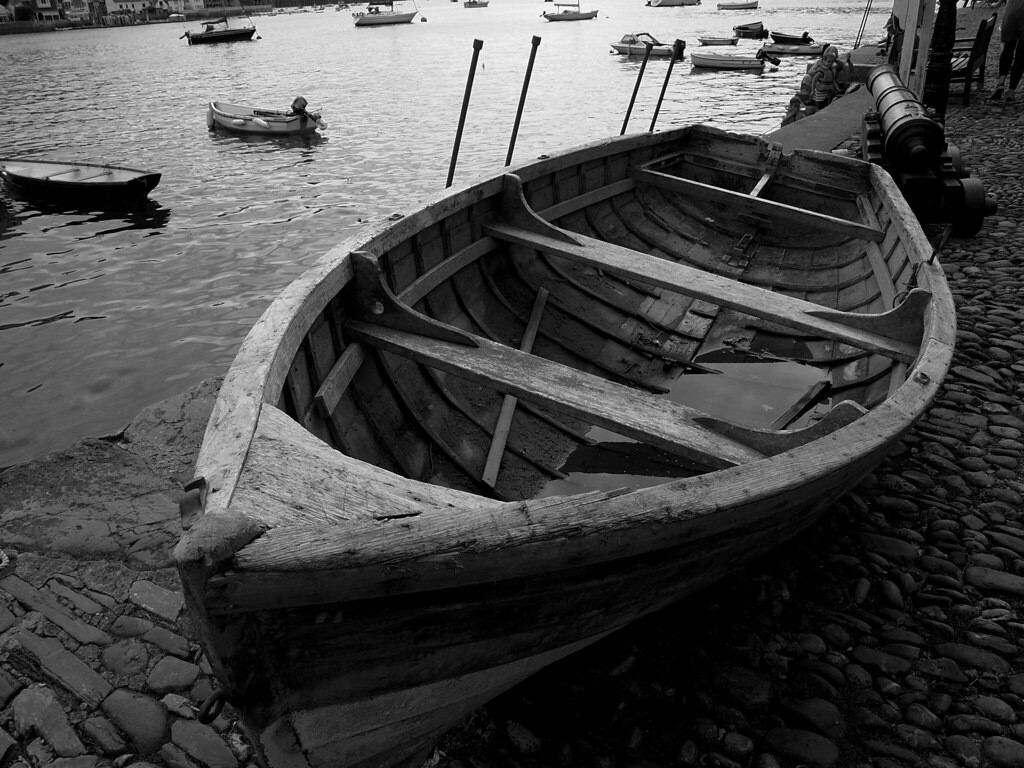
[990,0,1024,103]
[787,45,855,124]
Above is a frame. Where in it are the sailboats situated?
[355,0,418,25]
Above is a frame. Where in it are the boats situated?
[543,0,599,20]
[610,31,682,56]
[208,94,327,139]
[771,31,814,44]
[734,21,768,39]
[690,49,779,70]
[717,0,759,9]
[0,156,162,210]
[184,15,257,45]
[697,35,738,46]
[171,124,963,766]
[464,0,489,8]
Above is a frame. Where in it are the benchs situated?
[348,250,870,472]
[909,12,997,106]
[487,174,934,363]
